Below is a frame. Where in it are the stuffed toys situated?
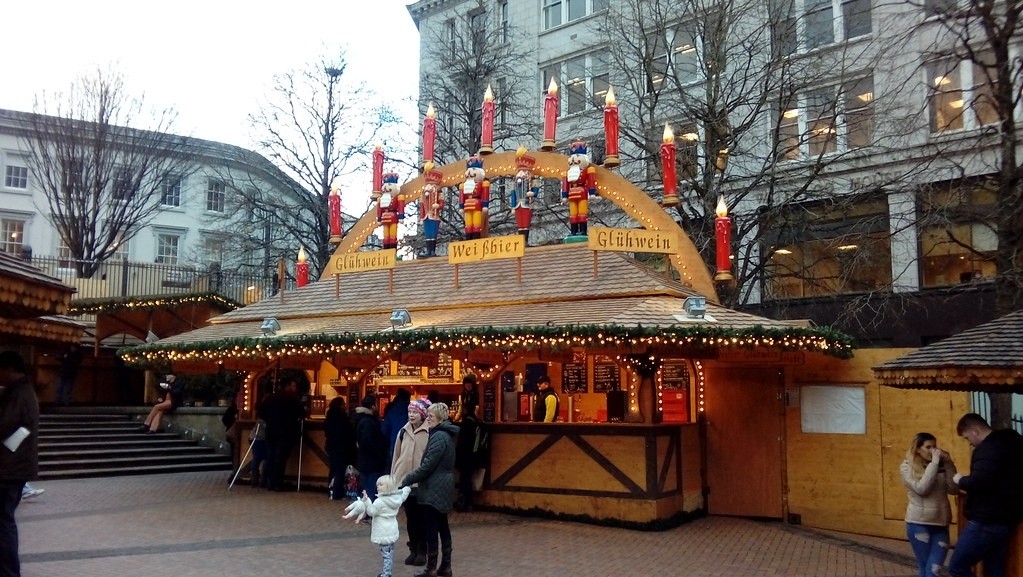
[341,500,365,524]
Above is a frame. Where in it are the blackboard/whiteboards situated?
[483,381,496,422]
[660,361,690,388]
[377,359,391,377]
[397,361,422,376]
[562,351,588,393]
[428,353,453,378]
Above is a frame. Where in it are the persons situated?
[0,351,39,577]
[323,397,349,499]
[531,375,560,422]
[459,157,490,240]
[455,400,487,512]
[454,373,479,421]
[238,379,305,491]
[222,396,238,462]
[382,391,411,458]
[561,142,597,236]
[347,393,382,523]
[949,414,1023,577]
[377,173,405,249]
[390,398,433,565]
[138,374,181,434]
[510,155,540,242]
[419,169,445,258]
[402,402,460,577]
[900,433,959,577]
[55,343,85,402]
[360,475,411,577]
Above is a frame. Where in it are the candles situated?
[329,181,342,244]
[478,83,495,155]
[603,85,621,167]
[662,120,681,206]
[419,101,436,172]
[370,136,385,200]
[297,245,310,288]
[715,194,732,280]
[541,76,559,150]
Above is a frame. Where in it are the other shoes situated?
[146,430,154,434]
[22,489,44,499]
[139,424,148,430]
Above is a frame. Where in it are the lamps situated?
[260,318,281,337]
[682,296,706,319]
[389,309,412,330]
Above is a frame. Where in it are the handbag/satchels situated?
[343,464,361,497]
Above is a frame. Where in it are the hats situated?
[408,398,432,419]
[534,375,549,383]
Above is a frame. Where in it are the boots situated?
[404,541,417,565]
[414,543,427,565]
[414,548,438,577]
[437,547,452,577]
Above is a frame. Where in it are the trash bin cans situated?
[593,355,621,393]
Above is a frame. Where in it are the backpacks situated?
[466,416,489,455]
